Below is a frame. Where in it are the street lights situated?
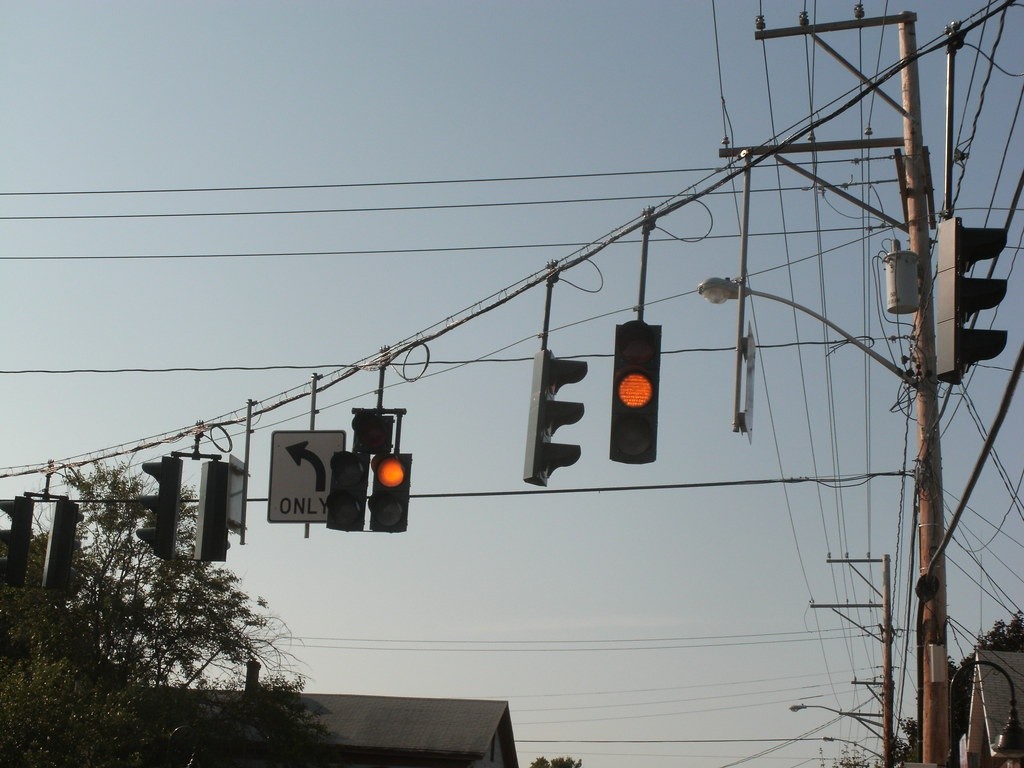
[700,275,951,768]
[947,659,1024,768]
[788,704,894,768]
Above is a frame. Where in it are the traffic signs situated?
[266,430,346,523]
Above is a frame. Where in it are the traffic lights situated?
[326,450,369,532]
[351,410,393,454]
[136,455,184,561]
[608,319,664,465]
[523,349,588,489]
[40,501,84,590]
[366,452,411,534]
[935,216,1008,384]
[0,495,35,587]
